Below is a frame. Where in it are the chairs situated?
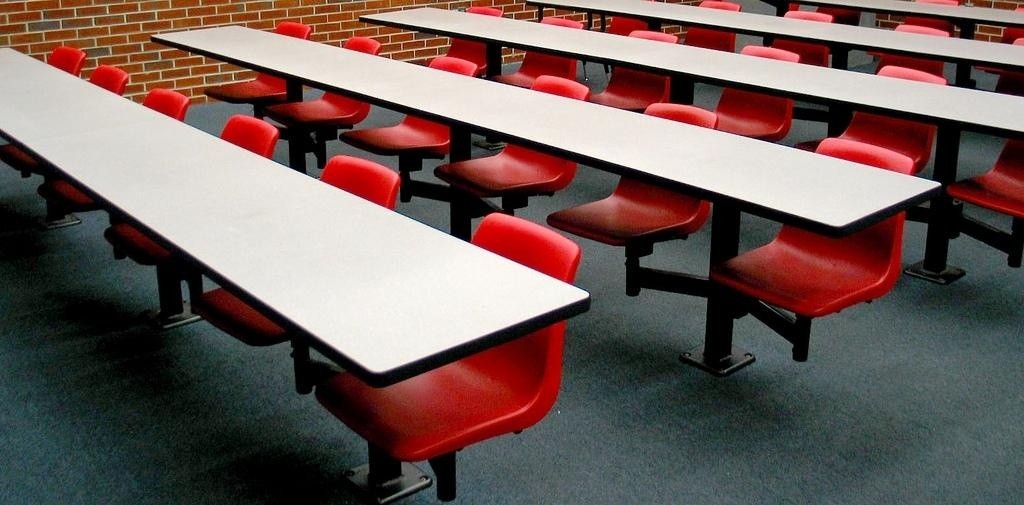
[0,61,134,175]
[101,113,282,272]
[37,85,190,211]
[795,66,947,176]
[193,153,405,348]
[810,0,860,26]
[491,17,584,95]
[866,24,949,74]
[994,4,1024,38]
[1001,39,1024,87]
[599,0,655,39]
[901,0,958,29]
[205,21,312,118]
[547,103,723,296]
[311,210,582,505]
[264,35,384,170]
[768,9,835,67]
[434,74,594,219]
[947,132,1024,265]
[339,57,483,201]
[592,32,678,111]
[38,44,87,75]
[428,3,503,73]
[711,44,803,140]
[677,2,739,55]
[711,134,920,364]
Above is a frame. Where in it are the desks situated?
[526,2,1024,90]
[812,1,1024,38]
[0,49,592,505]
[150,27,942,376]
[358,7,1024,287]
